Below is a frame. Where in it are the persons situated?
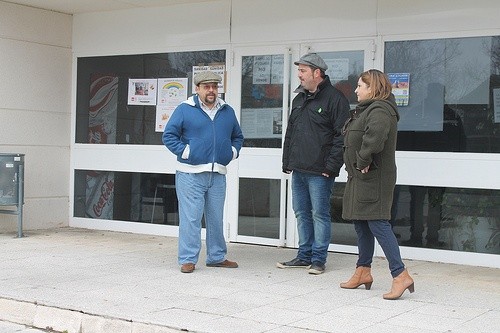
[162,71,244,272]
[275,52,350,275]
[400,84,467,248]
[340,67,415,299]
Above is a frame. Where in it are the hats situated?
[294,52,328,72]
[194,71,222,85]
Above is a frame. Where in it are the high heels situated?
[340,266,373,290]
[383,270,415,300]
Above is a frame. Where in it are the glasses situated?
[199,85,218,90]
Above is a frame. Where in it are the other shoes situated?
[308,262,326,274]
[276,258,311,269]
[180,263,196,273]
[206,260,238,268]
